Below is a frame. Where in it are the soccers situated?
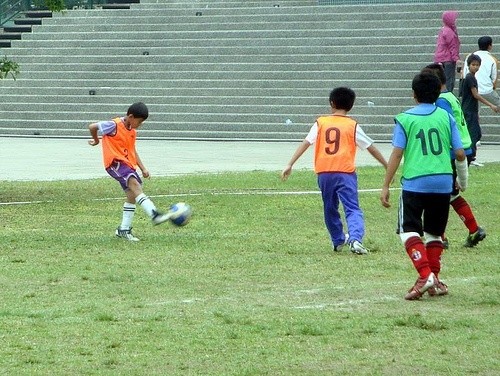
[168,201,192,227]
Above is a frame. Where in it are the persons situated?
[280,86,395,254]
[380,72,468,300]
[421,10,500,250]
[88,101,173,242]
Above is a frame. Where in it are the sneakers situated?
[350,239,368,255]
[332,233,349,251]
[404,272,438,300]
[428,279,448,296]
[442,238,448,249]
[115,227,139,241]
[151,209,173,226]
[463,228,486,247]
